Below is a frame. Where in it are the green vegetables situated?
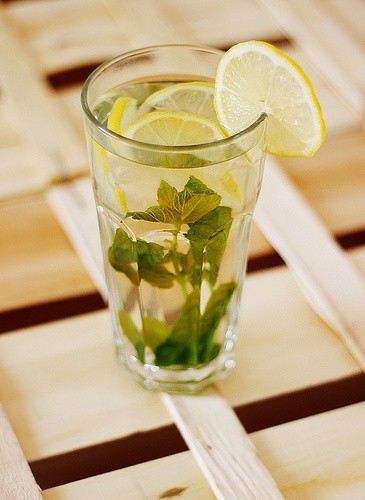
[107,154,238,369]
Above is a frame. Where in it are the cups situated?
[79,43,267,395]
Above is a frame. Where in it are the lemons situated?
[211,40,325,160]
[99,79,254,209]
